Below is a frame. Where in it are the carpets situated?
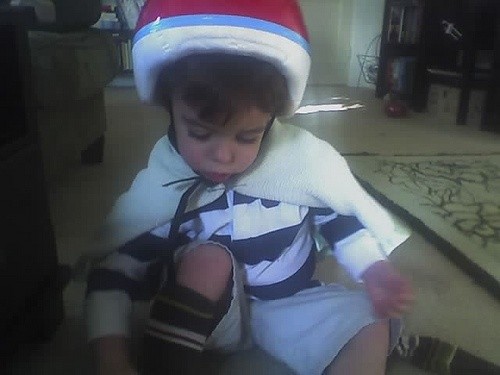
[336,152,500,302]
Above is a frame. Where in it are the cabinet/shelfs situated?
[376,1,475,113]
[0,6,65,375]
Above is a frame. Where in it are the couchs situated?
[0,0,117,170]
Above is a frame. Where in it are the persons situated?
[82,0,415,374]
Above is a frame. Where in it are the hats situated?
[132,0,312,121]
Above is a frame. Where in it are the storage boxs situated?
[429,76,487,125]
[407,64,444,102]
